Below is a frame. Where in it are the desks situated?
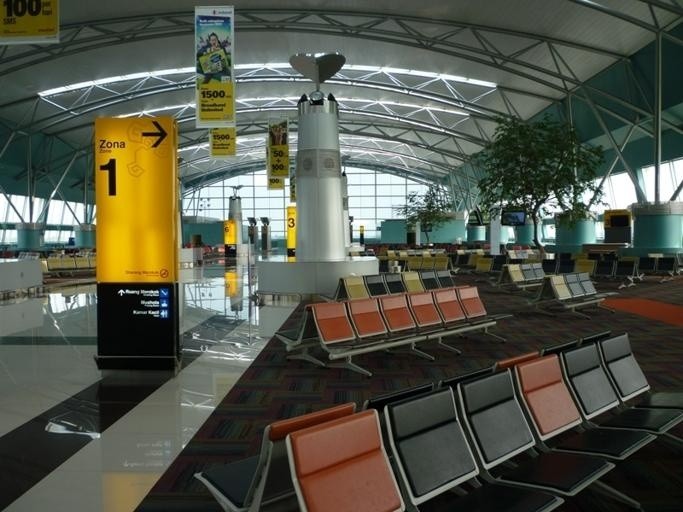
[0,258,43,292]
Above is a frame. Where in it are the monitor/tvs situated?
[421,223,432,232]
[501,210,525,226]
[611,215,628,226]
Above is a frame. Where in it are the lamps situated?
[310,91,324,103]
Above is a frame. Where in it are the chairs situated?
[0,242,215,277]
